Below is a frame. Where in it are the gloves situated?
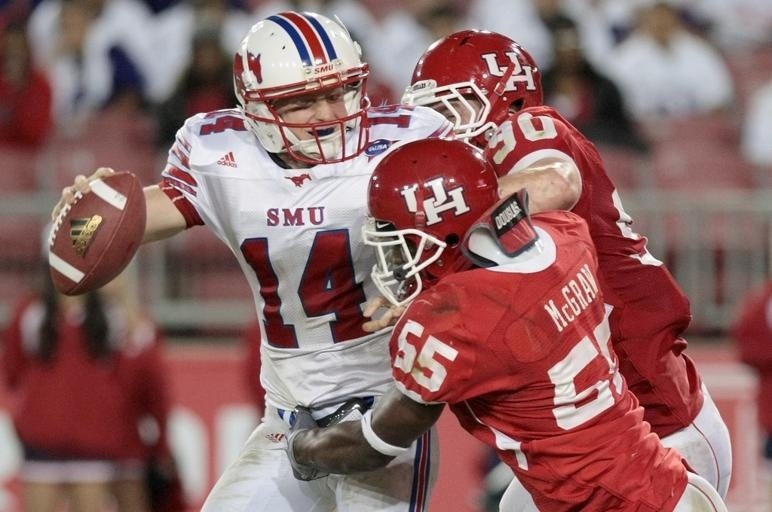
[284,405,329,482]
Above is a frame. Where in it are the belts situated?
[278,396,374,426]
[278,409,296,427]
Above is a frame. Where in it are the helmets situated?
[401,28,543,146]
[232,12,373,166]
[360,133,501,309]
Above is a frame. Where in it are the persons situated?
[2,0,772,511]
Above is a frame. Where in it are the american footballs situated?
[48,171,146,296]
